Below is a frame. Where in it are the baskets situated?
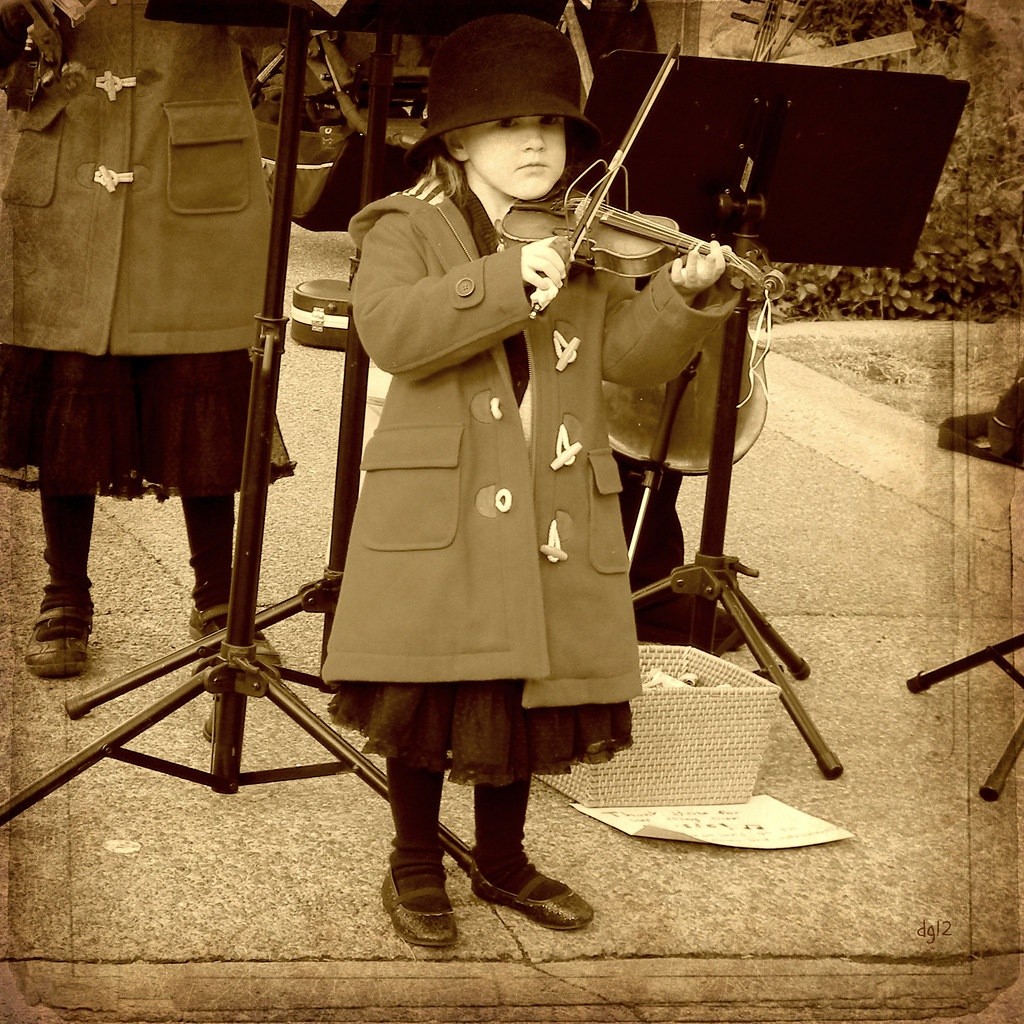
[535,643,783,808]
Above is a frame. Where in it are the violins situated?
[499,184,787,302]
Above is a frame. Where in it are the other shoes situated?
[189,601,281,668]
[470,855,595,930]
[23,602,96,678]
[381,864,459,946]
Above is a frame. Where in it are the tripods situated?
[0,0,571,881]
[575,49,967,783]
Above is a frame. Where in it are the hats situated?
[404,14,605,181]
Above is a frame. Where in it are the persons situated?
[0,1,303,677]
[323,13,742,950]
